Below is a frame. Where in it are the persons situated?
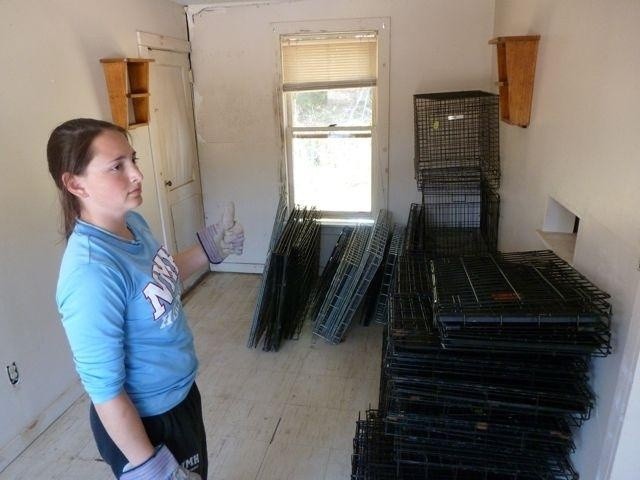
[47,117,246,478]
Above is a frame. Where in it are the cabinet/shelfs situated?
[99,58,153,132]
[488,37,540,129]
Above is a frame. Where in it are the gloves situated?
[196,200,244,264]
[116,444,202,480]
